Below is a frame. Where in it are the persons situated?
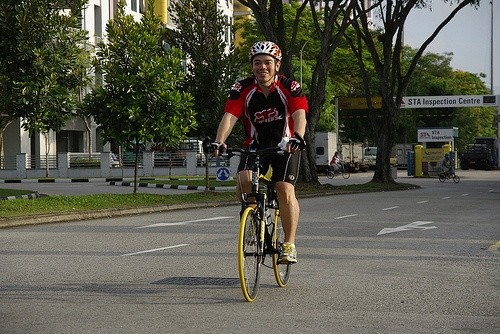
[212,41,309,266]
[439,154,455,176]
[330,151,345,173]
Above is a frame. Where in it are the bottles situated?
[265,205,272,234]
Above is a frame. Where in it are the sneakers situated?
[276,244,297,264]
[245,216,258,247]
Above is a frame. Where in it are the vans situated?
[358,146,377,172]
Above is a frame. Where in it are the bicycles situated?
[326,160,350,179]
[208,128,307,302]
[439,166,460,183]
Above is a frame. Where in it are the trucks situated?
[337,142,364,173]
[313,130,337,177]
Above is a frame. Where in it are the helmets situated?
[250,41,281,61]
